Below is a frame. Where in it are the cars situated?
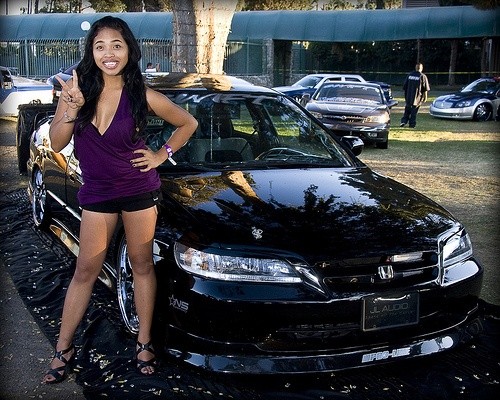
[24,72,485,377]
[270,74,398,150]
[47,59,82,92]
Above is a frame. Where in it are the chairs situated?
[188,102,255,162]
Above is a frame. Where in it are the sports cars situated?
[429,76,500,122]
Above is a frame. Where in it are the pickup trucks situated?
[0,61,56,117]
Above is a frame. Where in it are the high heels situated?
[43,343,77,384]
[136,339,160,376]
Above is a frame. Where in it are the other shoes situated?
[409,124,415,128]
[399,122,406,127]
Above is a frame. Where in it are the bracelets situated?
[64,112,78,122]
[162,144,177,166]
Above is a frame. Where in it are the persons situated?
[44,16,199,385]
[144,63,161,72]
[399,63,430,129]
